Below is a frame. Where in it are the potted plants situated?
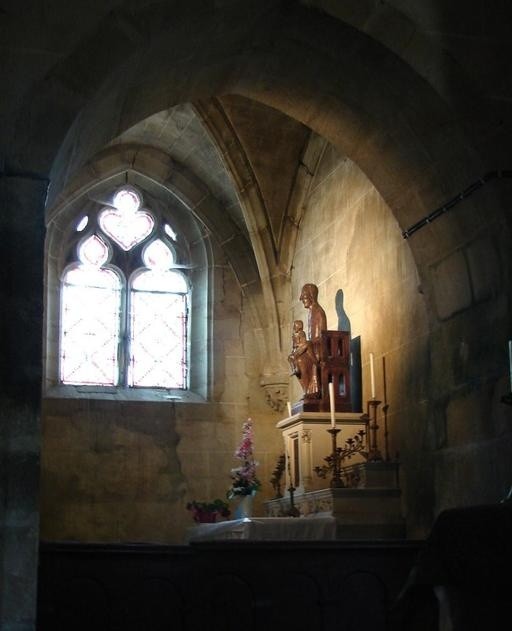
[187,499,231,525]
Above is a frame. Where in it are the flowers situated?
[227,417,261,496]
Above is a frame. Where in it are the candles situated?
[370,352,376,399]
[329,383,336,429]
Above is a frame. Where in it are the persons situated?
[288,320,308,377]
[295,279,327,397]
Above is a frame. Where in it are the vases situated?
[236,495,256,520]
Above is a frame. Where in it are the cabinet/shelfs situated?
[276,413,366,497]
